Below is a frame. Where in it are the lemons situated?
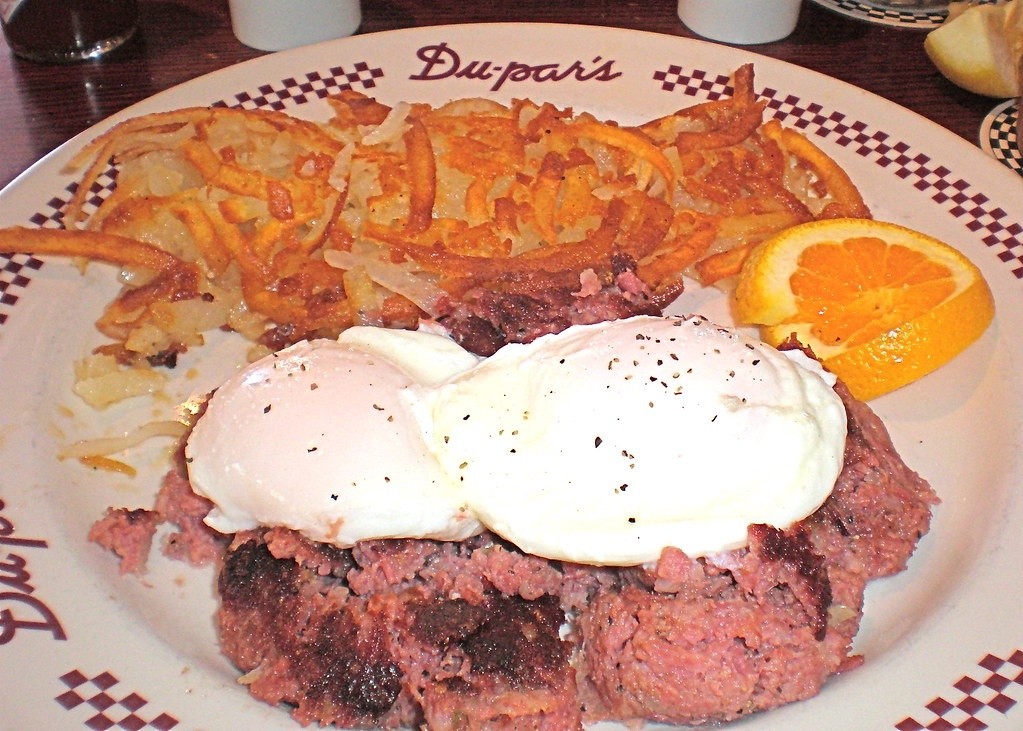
[923,0,1023,100]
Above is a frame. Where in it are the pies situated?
[1,88,874,324]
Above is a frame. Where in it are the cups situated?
[678,0,802,44]
[0,0,140,61]
[229,0,362,51]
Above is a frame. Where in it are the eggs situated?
[413,313,847,567]
[184,326,494,546]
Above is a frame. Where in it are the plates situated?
[812,0,943,32]
[979,97,1023,179]
[0,23,1023,731]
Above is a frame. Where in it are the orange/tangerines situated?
[733,217,997,405]
